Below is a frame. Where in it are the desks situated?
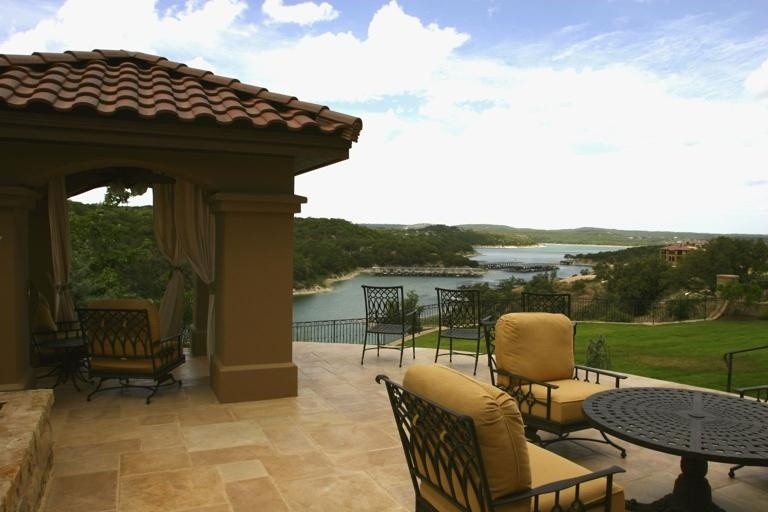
[580,386,767,511]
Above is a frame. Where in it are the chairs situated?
[31,319,94,392]
[73,299,186,405]
[521,291,577,338]
[375,362,627,512]
[435,287,490,376]
[361,284,417,368]
[483,311,627,458]
